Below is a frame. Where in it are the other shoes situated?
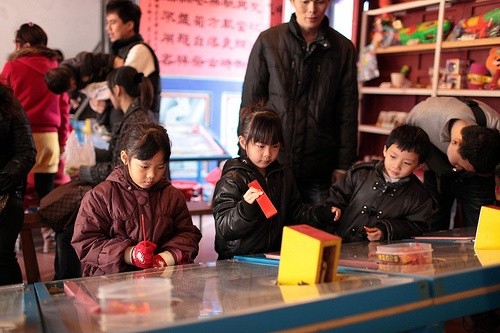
[43,238,56,253]
[16,237,21,249]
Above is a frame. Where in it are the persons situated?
[405,97,500,232]
[71,120,202,278]
[237,0,358,203]
[323,124,434,244]
[46,51,125,109]
[68,0,161,139]
[211,95,340,260]
[0,21,73,254]
[52,66,157,282]
[0,83,37,286]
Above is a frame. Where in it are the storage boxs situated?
[375,242,434,265]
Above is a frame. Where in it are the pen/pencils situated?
[141,214,146,246]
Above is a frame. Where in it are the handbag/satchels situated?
[38,181,92,233]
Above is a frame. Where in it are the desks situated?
[0,226,500,333]
[19,193,212,285]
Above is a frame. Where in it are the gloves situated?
[154,251,175,268]
[124,241,157,268]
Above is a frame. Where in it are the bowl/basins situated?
[97,277,175,313]
[376,242,434,268]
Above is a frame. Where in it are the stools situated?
[172,181,203,232]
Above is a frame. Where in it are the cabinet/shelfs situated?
[358,0,500,201]
